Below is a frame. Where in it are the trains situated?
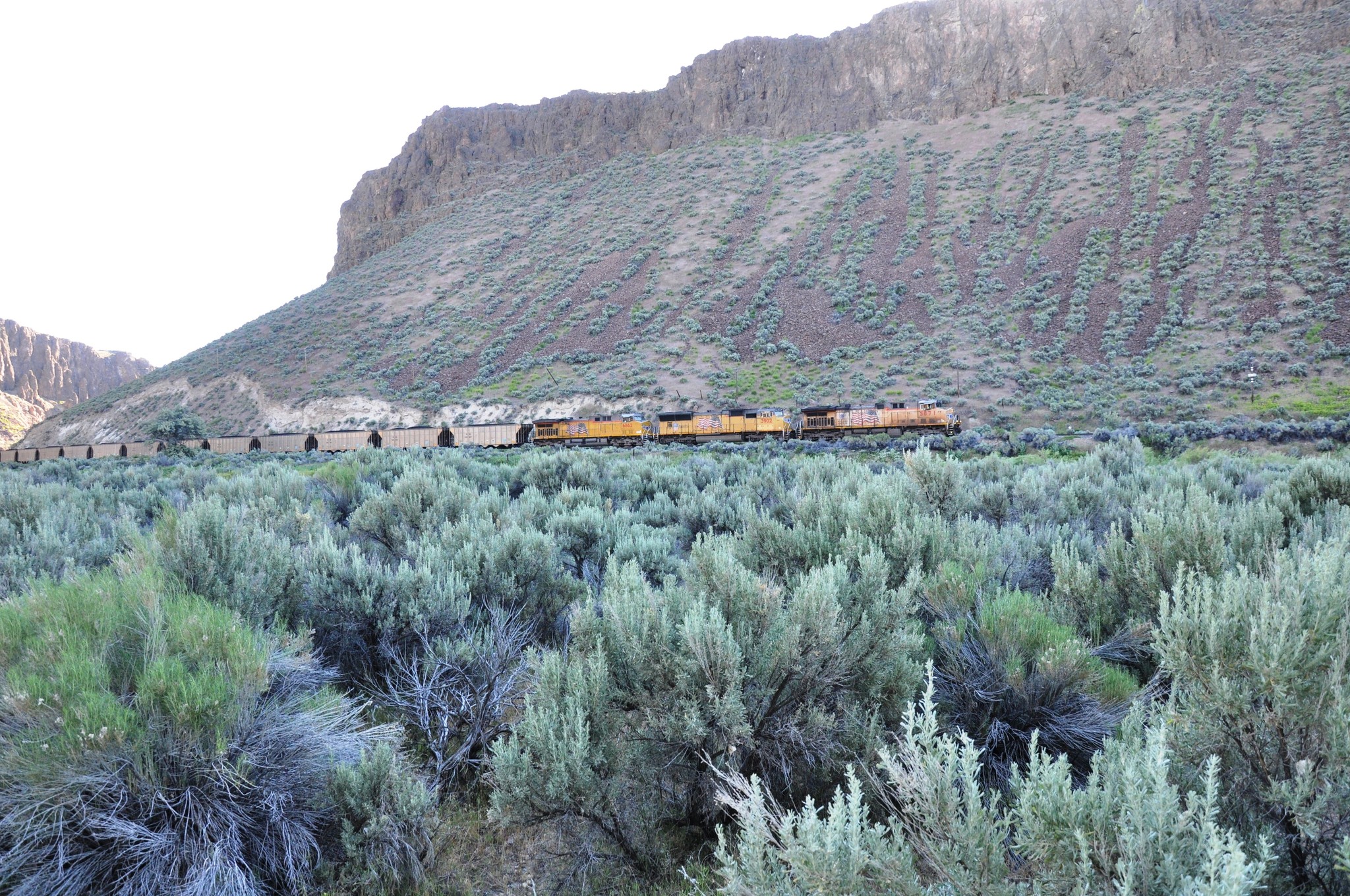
[0,397,963,465]
